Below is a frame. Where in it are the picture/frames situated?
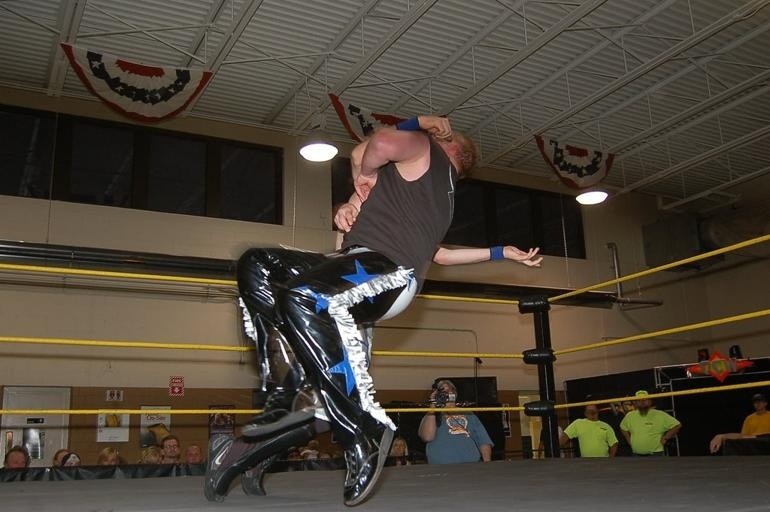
[208,404,236,439]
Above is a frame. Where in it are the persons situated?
[709,394,770,454]
[559,390,682,458]
[3,445,31,468]
[53,435,203,467]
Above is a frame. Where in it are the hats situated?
[635,390,648,395]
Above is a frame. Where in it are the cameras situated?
[435,384,457,405]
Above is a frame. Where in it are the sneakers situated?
[344,420,395,507]
[205,410,316,504]
[242,387,315,436]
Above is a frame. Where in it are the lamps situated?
[297,129,339,164]
